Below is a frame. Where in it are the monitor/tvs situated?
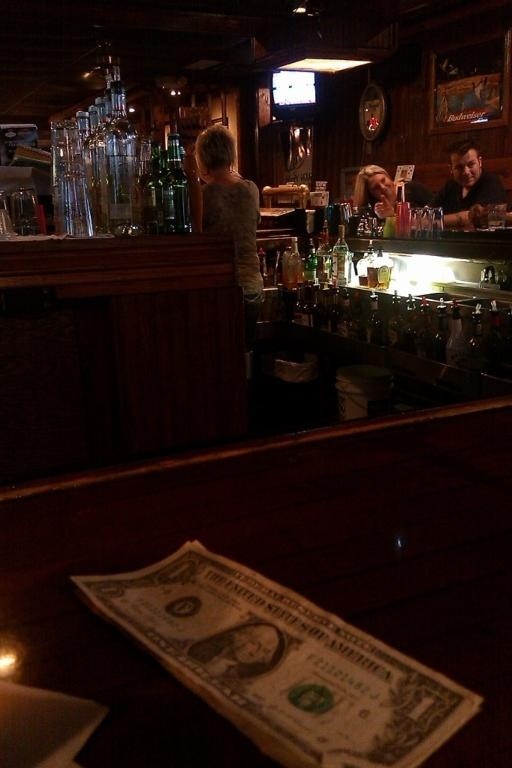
[268,68,322,121]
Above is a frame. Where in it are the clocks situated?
[358,84,388,141]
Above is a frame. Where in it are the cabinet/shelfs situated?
[257,284,512,403]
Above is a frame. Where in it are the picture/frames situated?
[427,28,509,135]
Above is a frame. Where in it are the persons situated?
[193,123,266,357]
[351,164,429,212]
[373,137,507,225]
[193,621,281,689]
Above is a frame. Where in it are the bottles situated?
[281,182,512,381]
[0,80,194,239]
